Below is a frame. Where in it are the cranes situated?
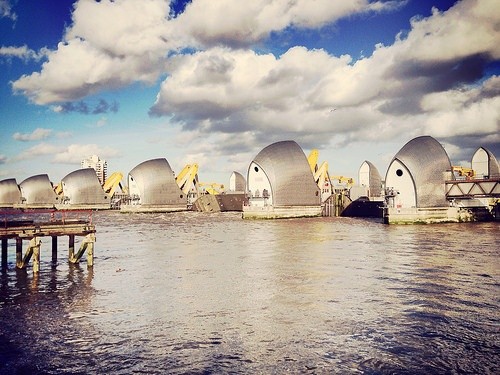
[176,164,202,195]
[101,168,128,196]
[308,150,353,196]
[452,165,476,181]
